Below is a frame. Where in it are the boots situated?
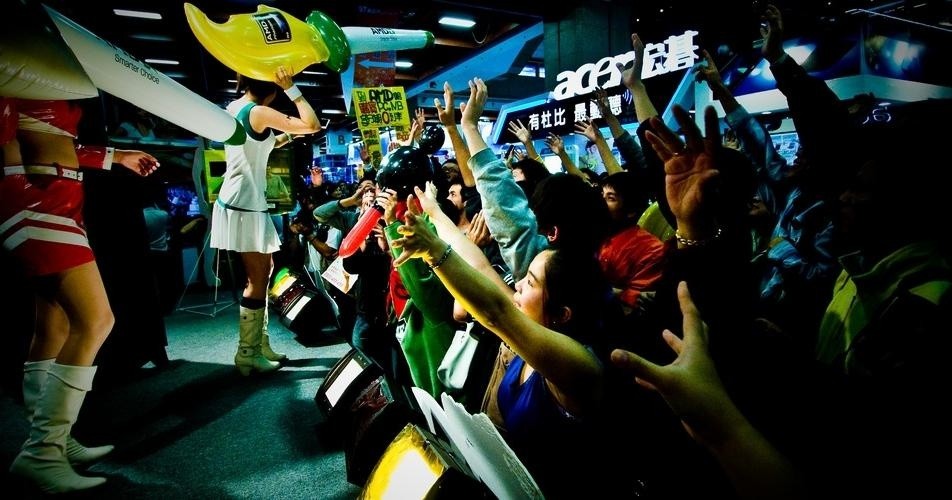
[202,262,223,287]
[232,304,281,379]
[262,296,288,365]
[19,356,117,473]
[9,360,110,498]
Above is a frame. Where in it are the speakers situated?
[296,138,312,156]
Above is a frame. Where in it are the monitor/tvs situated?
[770,130,799,166]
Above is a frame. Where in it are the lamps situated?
[269,270,305,308]
[312,347,387,425]
[279,287,326,348]
[356,422,492,500]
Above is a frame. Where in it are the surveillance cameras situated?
[472,24,488,43]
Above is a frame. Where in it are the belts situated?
[2,163,85,184]
